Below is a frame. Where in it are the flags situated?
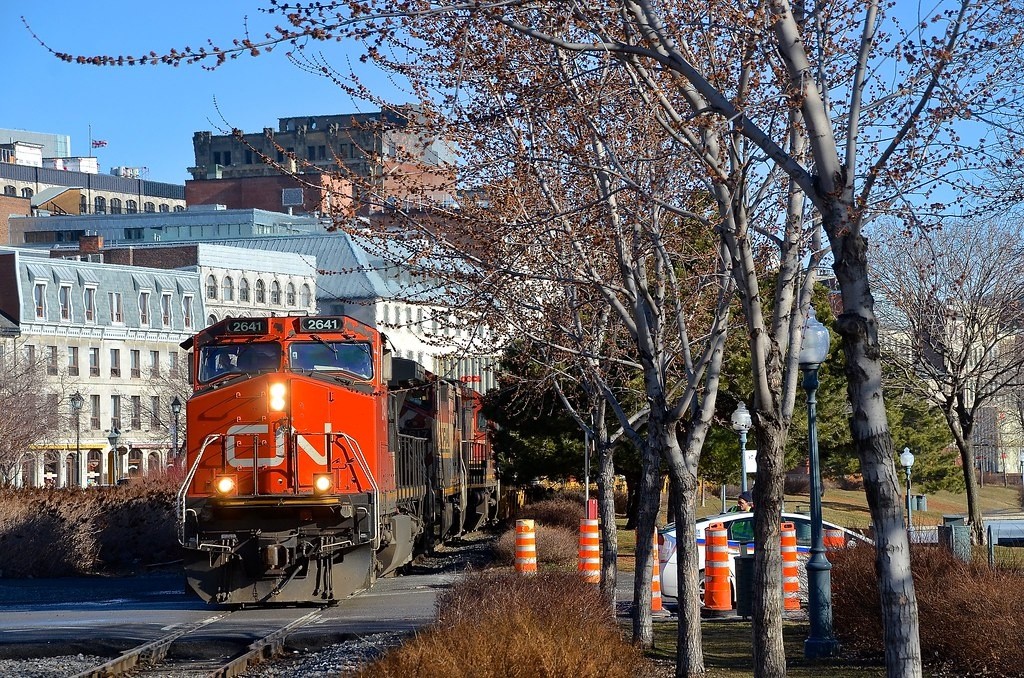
[92,140,107,147]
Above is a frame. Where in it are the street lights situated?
[731,400,752,493]
[108,427,121,487]
[900,447,915,547]
[70,387,85,488]
[796,303,843,662]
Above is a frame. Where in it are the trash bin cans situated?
[937,515,972,568]
[906,494,917,510]
[917,495,927,512]
[733,540,755,615]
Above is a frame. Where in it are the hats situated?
[738,490,752,502]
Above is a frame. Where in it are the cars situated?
[658,510,876,617]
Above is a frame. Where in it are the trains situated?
[174,309,526,610]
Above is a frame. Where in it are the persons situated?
[729,492,756,538]
